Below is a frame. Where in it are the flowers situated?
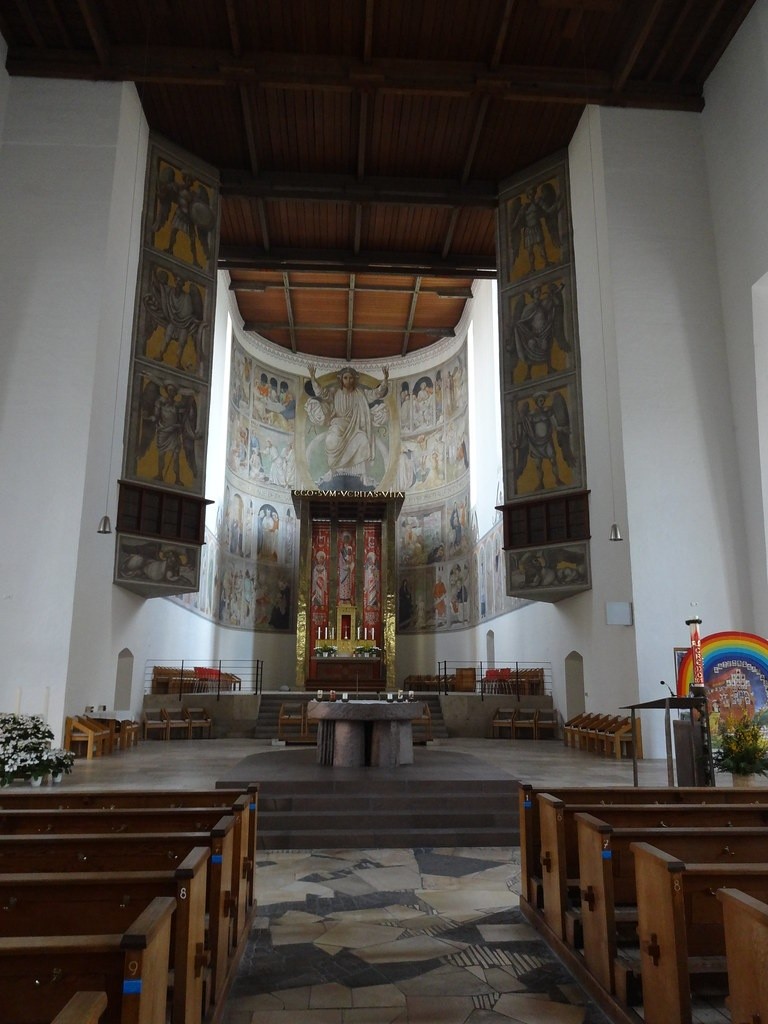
[355,645,365,654]
[314,646,323,653]
[328,646,338,653]
[712,702,768,778]
[0,711,76,788]
[368,646,382,654]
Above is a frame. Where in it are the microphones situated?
[661,681,674,697]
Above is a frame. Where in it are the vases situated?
[317,654,321,658]
[331,654,334,657]
[732,773,756,787]
[52,773,62,783]
[0,778,10,787]
[372,654,376,657]
[30,776,43,787]
[358,654,362,657]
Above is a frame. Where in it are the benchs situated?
[516,780,768,1024]
[0,780,260,1024]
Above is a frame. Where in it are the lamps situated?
[97,107,145,534]
[587,102,624,541]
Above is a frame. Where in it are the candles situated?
[365,628,368,640]
[357,627,360,640]
[318,626,321,639]
[372,627,375,640]
[325,627,328,639]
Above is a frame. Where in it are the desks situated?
[86,711,136,752]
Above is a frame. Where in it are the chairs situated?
[305,710,319,736]
[411,701,431,739]
[141,704,170,741]
[64,714,140,760]
[473,667,544,695]
[185,706,212,740]
[277,702,304,738]
[490,707,644,761]
[165,705,191,740]
[403,674,457,692]
[149,665,242,694]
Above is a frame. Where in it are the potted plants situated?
[321,644,335,658]
[364,648,371,657]
